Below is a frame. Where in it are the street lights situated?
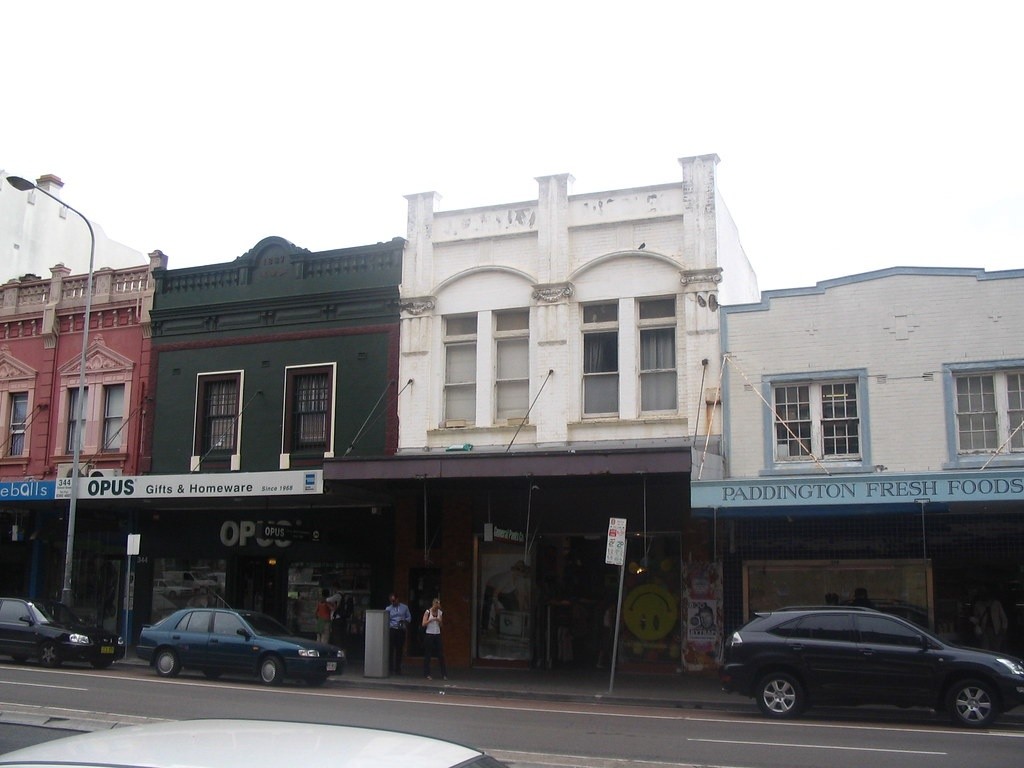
[7,177,94,607]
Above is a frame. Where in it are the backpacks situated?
[333,593,354,621]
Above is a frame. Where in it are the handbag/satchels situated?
[970,617,982,635]
[416,623,427,642]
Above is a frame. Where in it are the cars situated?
[136,608,344,685]
[0,597,125,668]
[153,571,225,599]
[716,606,1024,727]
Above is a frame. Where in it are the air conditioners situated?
[55,464,86,478]
[90,469,120,481]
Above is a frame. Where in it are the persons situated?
[421,598,450,681]
[311,587,337,645]
[491,558,527,617]
[690,602,717,635]
[844,588,877,610]
[326,585,349,650]
[824,592,839,605]
[968,580,1009,652]
[384,591,411,676]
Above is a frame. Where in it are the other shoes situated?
[426,674,432,680]
[442,675,448,681]
[395,670,401,675]
[596,664,605,669]
